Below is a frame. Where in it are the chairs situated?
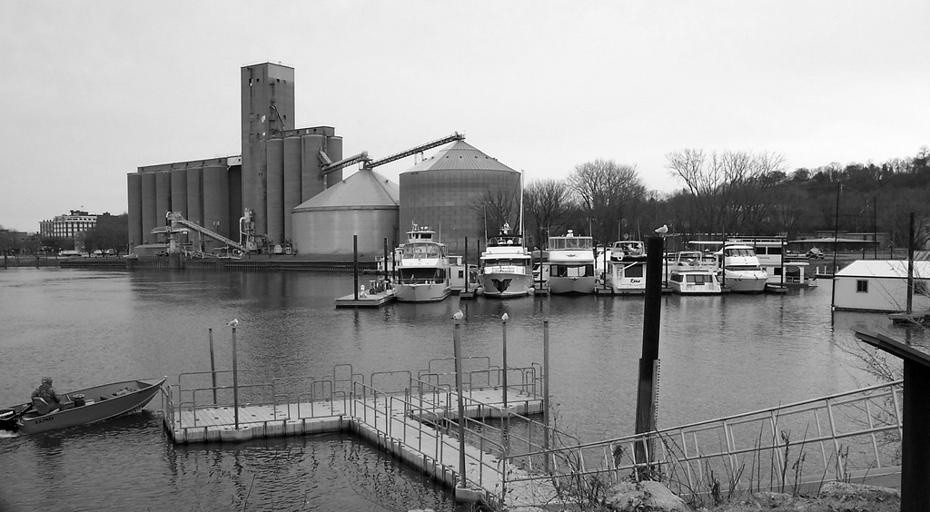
[32,396,59,415]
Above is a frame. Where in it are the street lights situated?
[452,309,467,486]
[230,319,241,428]
[500,312,509,407]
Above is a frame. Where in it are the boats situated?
[475,167,534,300]
[387,219,470,305]
[1,375,169,437]
[535,216,817,296]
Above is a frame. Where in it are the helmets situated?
[41,377,52,385]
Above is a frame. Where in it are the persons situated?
[30,375,66,415]
